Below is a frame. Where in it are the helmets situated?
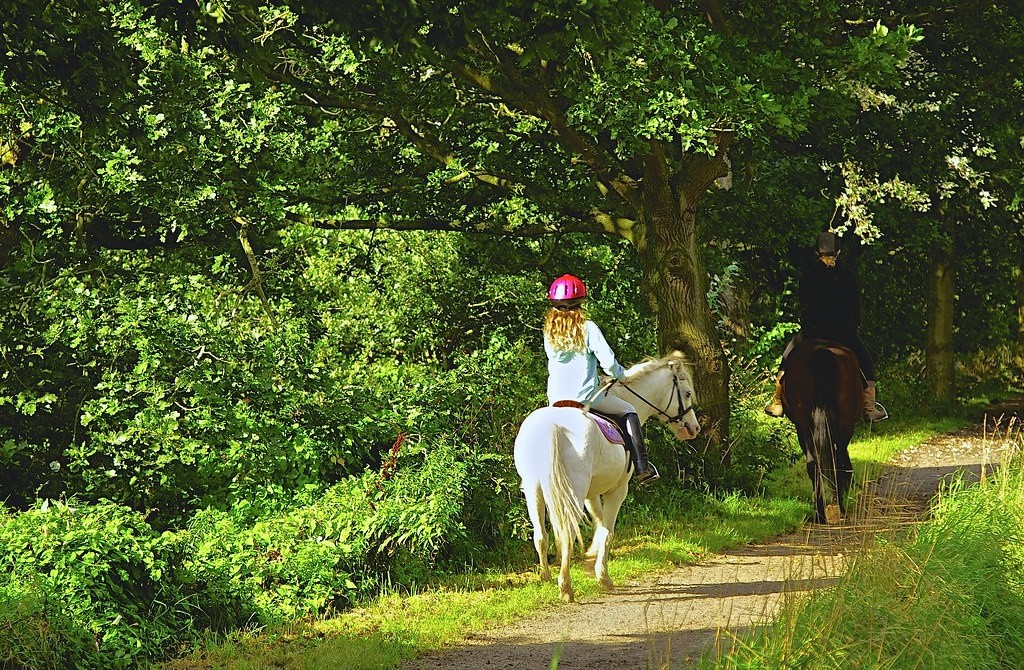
[548,273,588,302]
[815,232,841,255]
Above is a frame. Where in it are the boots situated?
[619,412,655,481]
[862,380,886,422]
[763,369,786,418]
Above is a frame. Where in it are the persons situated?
[542,274,660,483]
[765,233,885,422]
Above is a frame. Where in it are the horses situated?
[512,348,702,605]
[777,339,865,525]
